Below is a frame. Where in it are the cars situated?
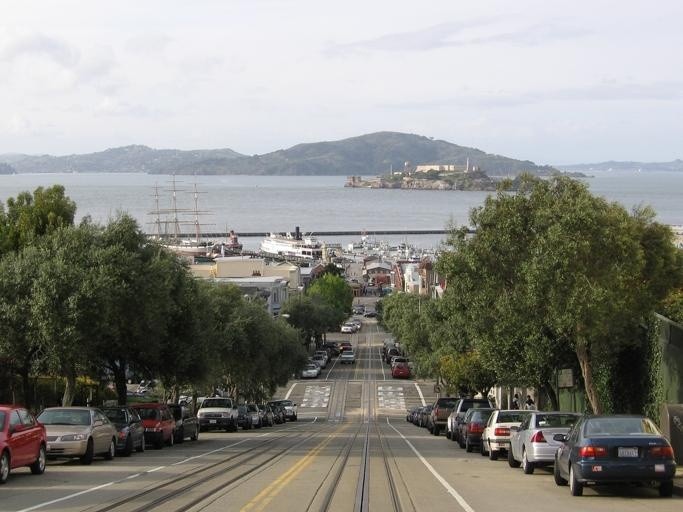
[507,412,584,474]
[35,406,118,464]
[124,367,298,443]
[123,402,176,450]
[404,395,495,456]
[341,303,381,333]
[554,415,675,497]
[382,337,416,382]
[0,403,48,485]
[480,409,534,460]
[97,404,146,456]
[296,339,357,379]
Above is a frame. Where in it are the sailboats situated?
[138,173,243,262]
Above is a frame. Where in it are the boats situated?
[340,232,454,264]
[259,227,332,262]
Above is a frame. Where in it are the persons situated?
[511,393,519,410]
[524,394,534,410]
[227,231,236,243]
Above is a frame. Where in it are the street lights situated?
[272,314,292,365]
[296,285,306,328]
[397,290,421,319]
